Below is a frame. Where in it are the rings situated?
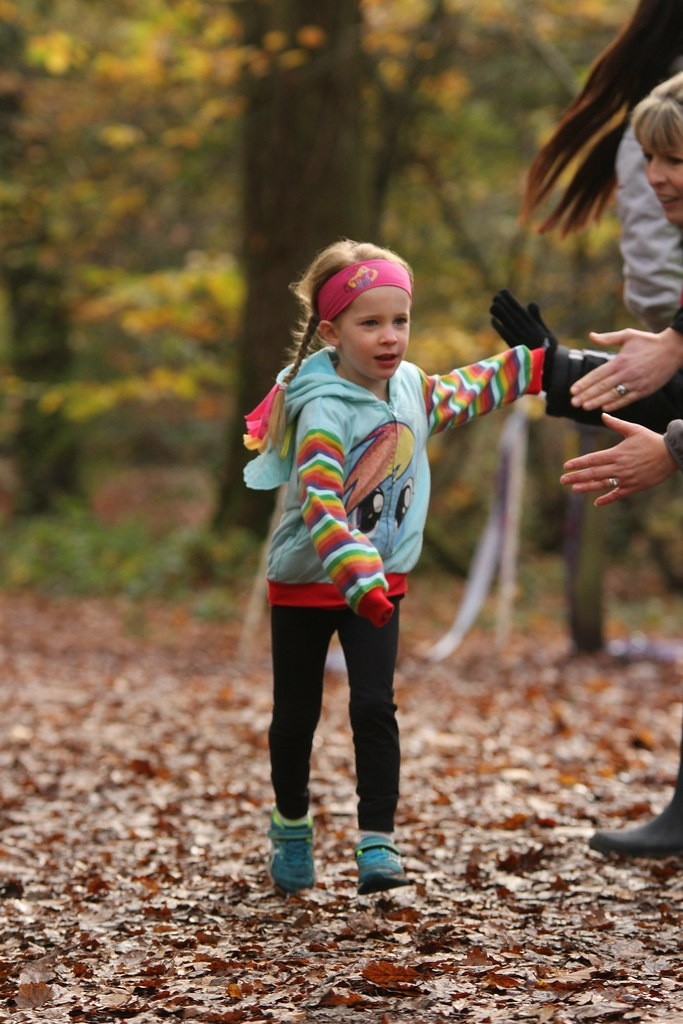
[609,478,617,490]
[616,384,628,395]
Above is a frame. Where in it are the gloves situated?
[489,288,558,392]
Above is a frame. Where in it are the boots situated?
[587,735,683,866]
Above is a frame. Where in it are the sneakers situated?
[353,833,410,895]
[266,805,315,895]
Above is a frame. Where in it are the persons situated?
[524,0,683,334]
[488,66,683,432]
[561,303,683,859]
[243,238,544,895]
